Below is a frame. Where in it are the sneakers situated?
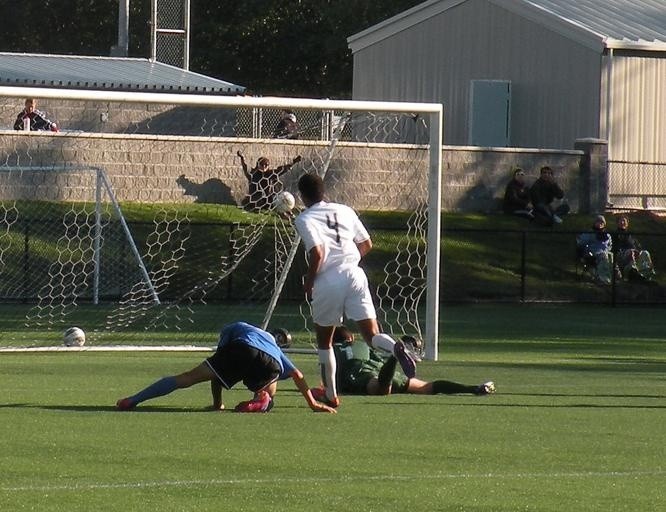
[393,338,416,378]
[116,398,131,410]
[477,381,496,396]
[234,400,264,412]
[552,214,563,224]
[407,349,422,362]
[311,387,339,407]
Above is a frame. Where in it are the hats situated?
[594,214,607,226]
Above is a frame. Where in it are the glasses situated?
[516,173,524,177]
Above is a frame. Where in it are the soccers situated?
[274,191,295,212]
[64,328,85,347]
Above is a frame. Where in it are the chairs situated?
[575,238,598,279]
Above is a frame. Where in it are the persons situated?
[236,149,304,217]
[501,167,553,229]
[574,214,623,287]
[317,320,498,406]
[614,214,657,287]
[272,109,300,140]
[113,320,339,416]
[13,97,55,132]
[527,164,571,225]
[289,171,417,406]
